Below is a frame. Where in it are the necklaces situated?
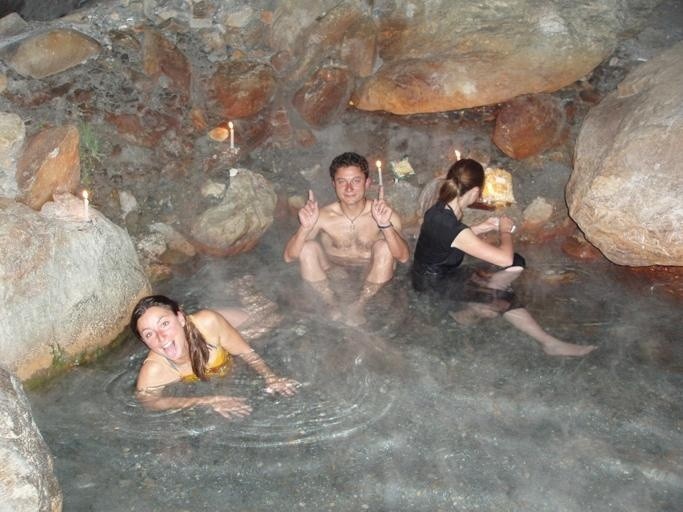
[446,204,452,211]
[339,198,365,224]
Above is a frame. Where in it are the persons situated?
[283,152,410,327]
[411,158,598,357]
[129,274,302,422]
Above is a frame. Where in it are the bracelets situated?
[377,223,393,228]
